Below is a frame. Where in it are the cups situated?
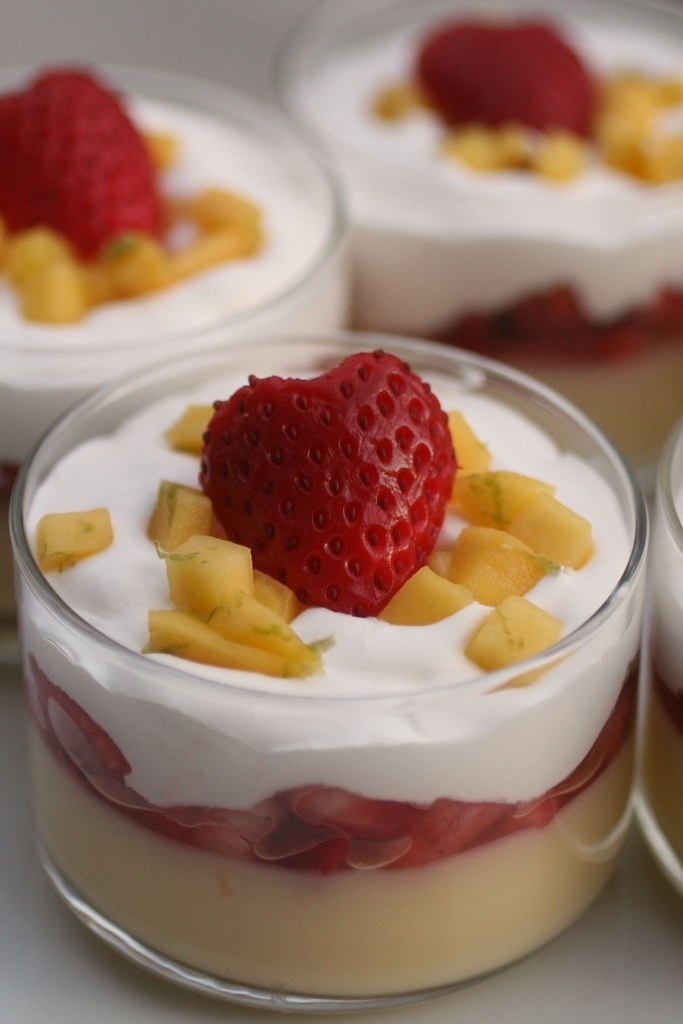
[0,61,354,469]
[14,335,651,1014]
[631,417,683,889]
[270,0,683,466]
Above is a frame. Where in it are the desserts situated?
[0,13,682,1000]
